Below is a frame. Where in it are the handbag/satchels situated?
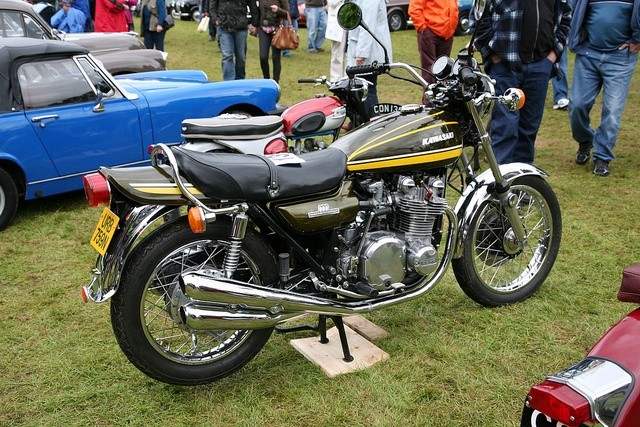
[271,11,299,50]
[146,0,174,31]
[198,16,209,33]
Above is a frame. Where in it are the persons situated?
[325,0,392,132]
[210,0,259,79]
[305,1,328,52]
[473,0,572,212]
[94,1,128,32]
[408,0,460,106]
[50,0,90,33]
[281,0,301,57]
[201,0,216,42]
[569,0,637,175]
[140,0,169,51]
[250,0,291,84]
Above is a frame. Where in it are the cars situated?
[0,0,169,75]
[378,0,474,32]
[172,0,203,21]
[295,0,327,27]
[0,37,283,231]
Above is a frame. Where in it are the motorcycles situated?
[180,74,373,155]
[517,263,640,427]
[80,2,563,384]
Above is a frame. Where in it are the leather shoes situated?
[515,190,532,204]
[317,48,324,51]
[310,50,319,53]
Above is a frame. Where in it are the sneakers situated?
[594,160,609,176]
[576,129,596,165]
[553,99,569,110]
